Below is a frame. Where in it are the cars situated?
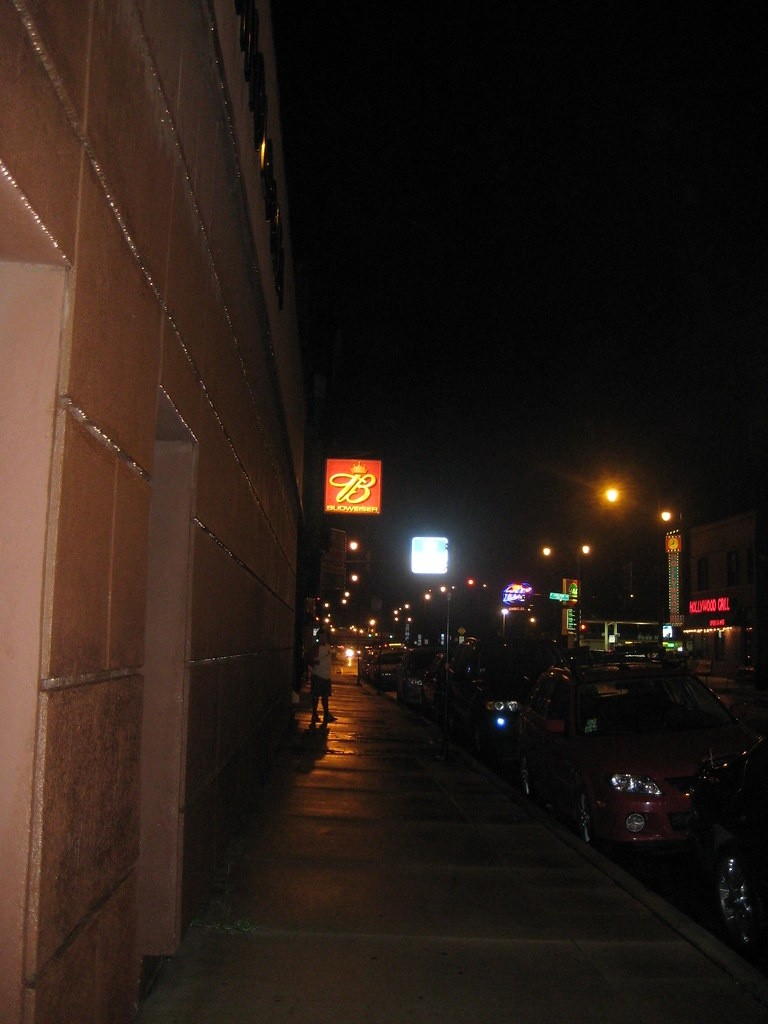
[356,632,590,768]
[691,730,768,958]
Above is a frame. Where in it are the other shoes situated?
[324,714,337,721]
[311,716,322,722]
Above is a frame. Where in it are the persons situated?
[304,628,338,722]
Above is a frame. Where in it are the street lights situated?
[406,529,454,759]
[576,543,593,648]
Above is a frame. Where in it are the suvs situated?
[510,648,766,856]
